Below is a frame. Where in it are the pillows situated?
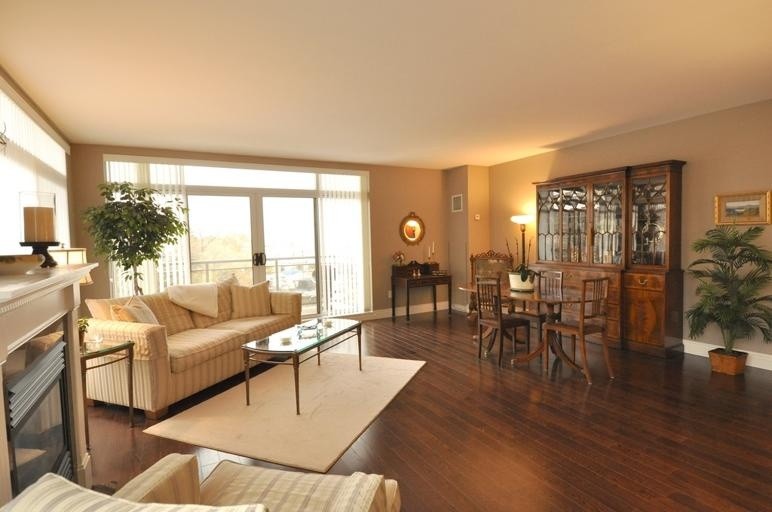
[85,273,273,324]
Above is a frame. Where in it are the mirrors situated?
[399,211,427,245]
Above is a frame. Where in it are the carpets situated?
[141,350,429,475]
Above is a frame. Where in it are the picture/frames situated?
[715,191,770,225]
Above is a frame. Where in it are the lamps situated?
[19,192,61,268]
[511,214,530,312]
[48,243,95,286]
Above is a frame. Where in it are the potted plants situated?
[686,225,772,376]
[77,317,90,349]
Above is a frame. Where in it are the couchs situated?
[84,272,302,420]
[0,451,407,512]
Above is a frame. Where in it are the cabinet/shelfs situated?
[531,159,685,359]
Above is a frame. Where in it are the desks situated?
[391,260,452,321]
[458,282,609,374]
[88,339,136,451]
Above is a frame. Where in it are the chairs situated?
[545,277,615,385]
[477,275,531,368]
[470,249,514,313]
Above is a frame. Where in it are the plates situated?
[1,254,45,274]
